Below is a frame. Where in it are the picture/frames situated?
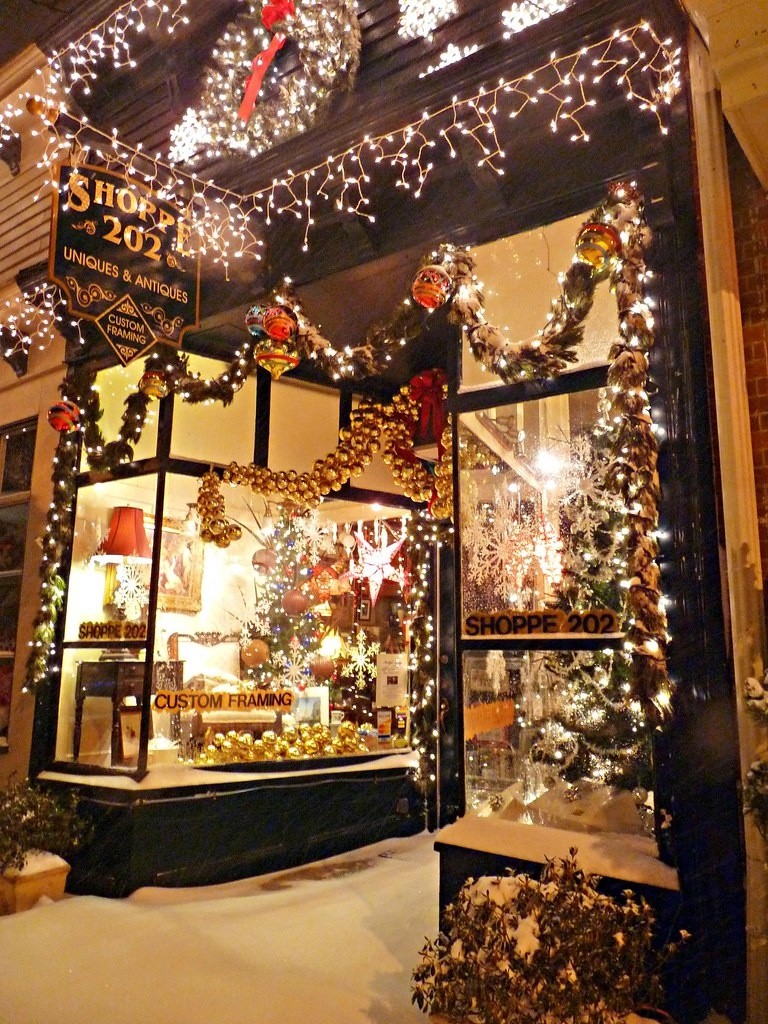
[104,515,206,614]
[283,686,330,726]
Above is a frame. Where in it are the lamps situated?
[95,507,155,660]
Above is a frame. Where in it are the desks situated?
[74,660,187,758]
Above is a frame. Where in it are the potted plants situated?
[0,770,95,916]
